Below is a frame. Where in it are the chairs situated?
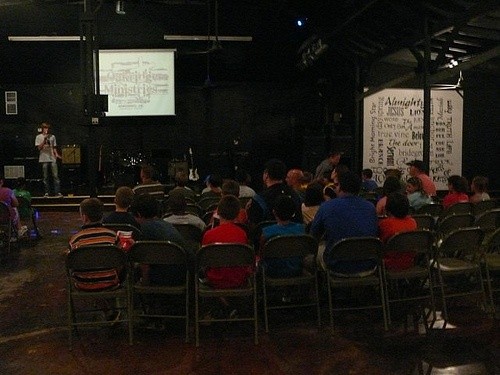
[67,185,500,346]
[0,196,32,257]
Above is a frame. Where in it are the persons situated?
[35,123,63,197]
[0,174,28,243]
[12,176,34,218]
[69,152,491,332]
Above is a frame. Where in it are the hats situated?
[406,159,427,171]
